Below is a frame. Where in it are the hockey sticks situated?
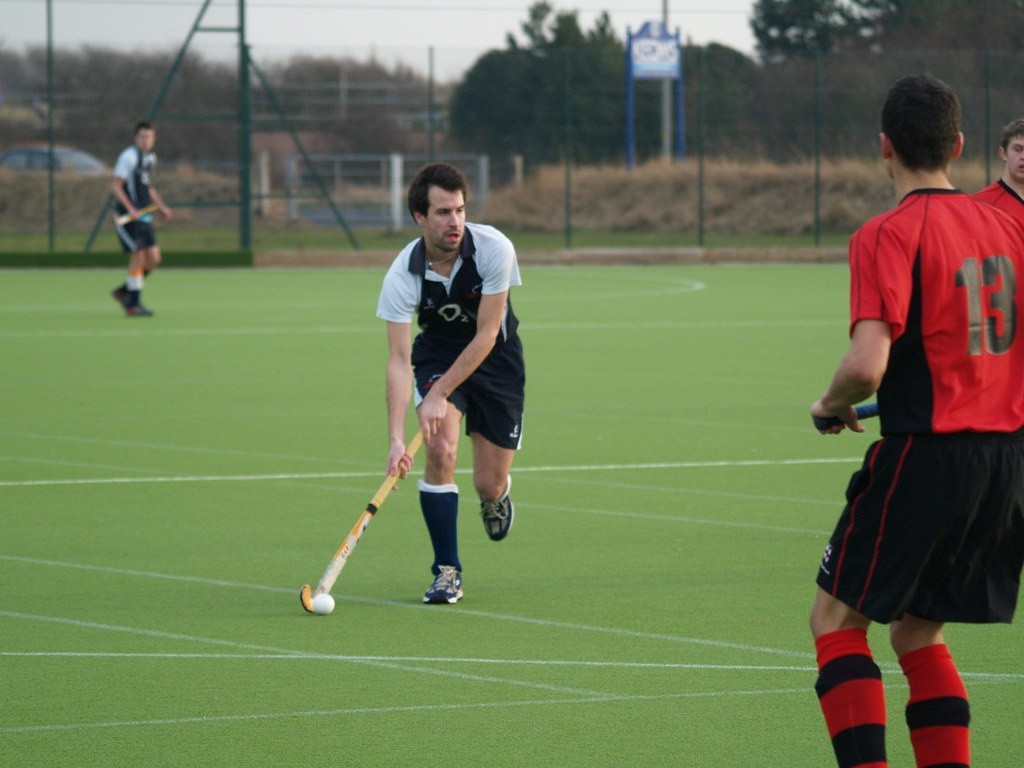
[812,400,878,434]
[111,202,156,253]
[298,428,424,613]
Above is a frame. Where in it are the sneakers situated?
[421,564,464,603]
[111,284,154,317]
[479,474,513,541]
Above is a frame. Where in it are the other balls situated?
[312,593,335,613]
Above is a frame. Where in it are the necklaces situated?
[426,253,454,265]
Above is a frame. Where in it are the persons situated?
[807,73,1024,768]
[970,117,1024,230]
[377,162,527,606]
[107,118,175,317]
